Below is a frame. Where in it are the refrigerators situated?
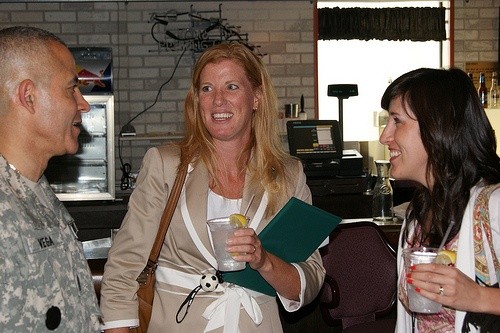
[41,47,115,203]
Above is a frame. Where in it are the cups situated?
[402,247,447,314]
[284,104,298,118]
[206,217,251,272]
[110,229,118,244]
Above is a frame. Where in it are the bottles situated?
[489,71,500,109]
[477,72,488,108]
[467,72,473,83]
[372,159,393,222]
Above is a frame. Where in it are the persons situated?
[99,40,327,333]
[0,25,102,333]
[379,67,500,333]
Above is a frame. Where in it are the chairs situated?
[318,221,398,330]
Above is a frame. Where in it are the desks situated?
[340,215,403,253]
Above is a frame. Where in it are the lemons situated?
[229,214,246,229]
[436,251,456,265]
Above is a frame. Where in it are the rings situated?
[252,246,256,254]
[438,284,443,294]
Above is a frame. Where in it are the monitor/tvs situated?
[287,118,344,161]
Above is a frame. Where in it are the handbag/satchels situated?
[133,269,156,333]
[461,183,500,333]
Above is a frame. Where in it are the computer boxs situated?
[302,149,363,179]
